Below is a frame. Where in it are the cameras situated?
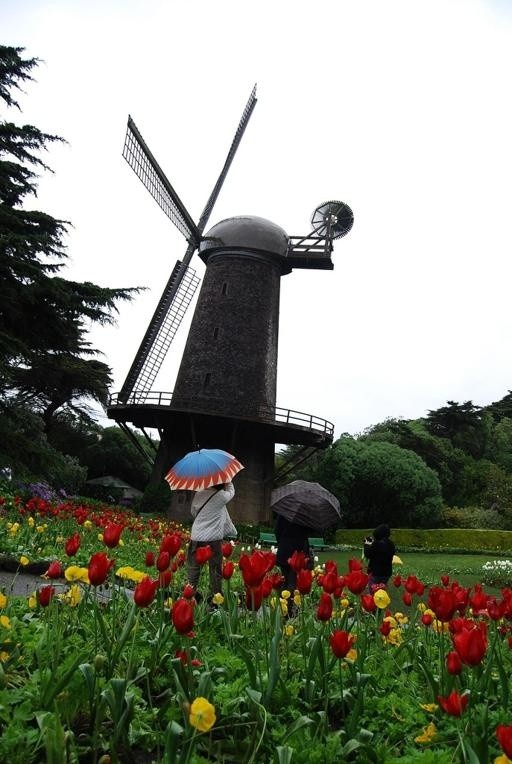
[366,537,372,542]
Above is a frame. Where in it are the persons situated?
[188,483,238,594]
[274,515,314,619]
[364,524,396,616]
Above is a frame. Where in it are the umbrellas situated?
[164,449,245,492]
[270,480,341,531]
